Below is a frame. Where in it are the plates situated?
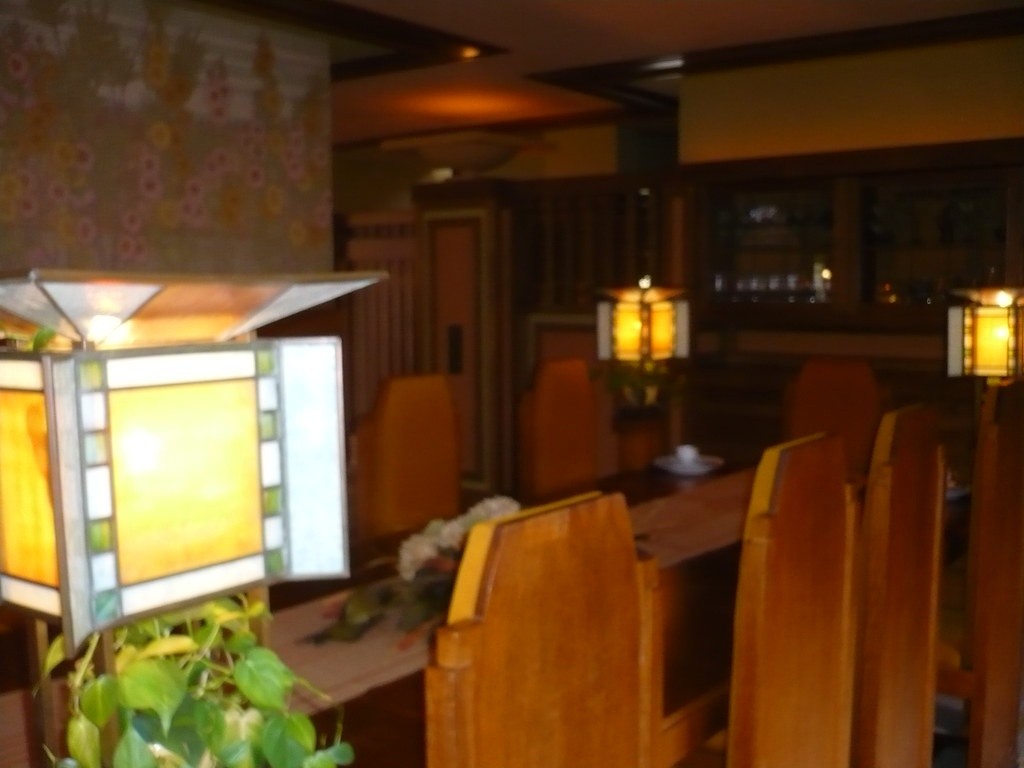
[655,457,725,477]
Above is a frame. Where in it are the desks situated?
[1,442,862,768]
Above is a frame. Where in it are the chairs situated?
[381,351,1023,767]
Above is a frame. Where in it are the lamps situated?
[945,284,1024,387]
[0,267,387,768]
[595,281,691,414]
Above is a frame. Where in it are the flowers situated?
[302,494,517,650]
